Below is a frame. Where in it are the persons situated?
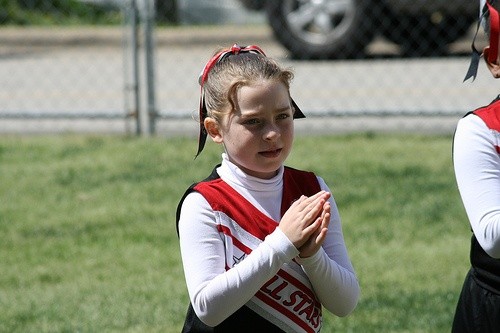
[450,0,500,333]
[175,44,361,333]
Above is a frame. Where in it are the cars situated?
[239,0,481,58]
